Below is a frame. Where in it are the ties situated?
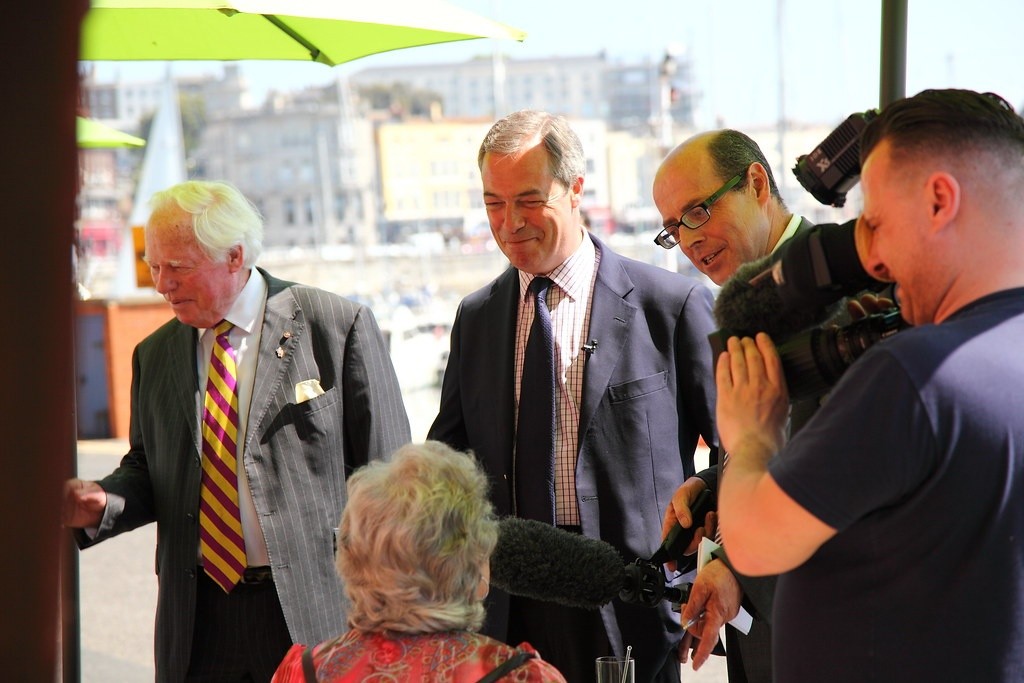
[200,319,249,594]
[514,276,558,531]
[715,452,730,546]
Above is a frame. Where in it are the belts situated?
[195,571,273,586]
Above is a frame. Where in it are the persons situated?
[271,438,566,683]
[425,110,718,683]
[69,178,411,683]
[654,127,896,683]
[717,88,1024,683]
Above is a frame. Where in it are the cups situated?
[596,656,636,683]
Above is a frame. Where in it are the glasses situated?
[654,173,744,249]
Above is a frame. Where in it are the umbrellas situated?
[78,117,145,149]
[80,1,488,68]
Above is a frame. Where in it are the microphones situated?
[488,516,626,608]
[712,254,792,342]
